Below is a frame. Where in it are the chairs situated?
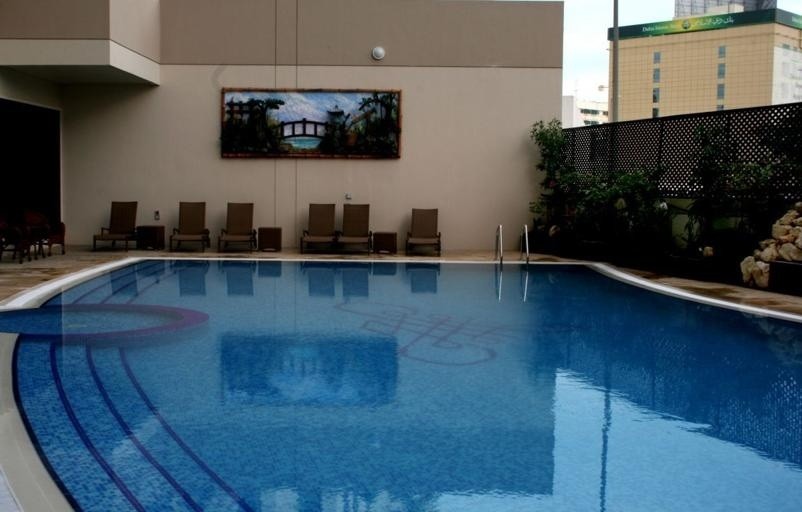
[216,263,258,296]
[340,265,371,296]
[108,265,137,299]
[169,262,209,297]
[169,202,210,253]
[301,264,336,296]
[338,205,370,255]
[1,211,67,262]
[300,203,337,252]
[219,203,257,250]
[406,207,442,252]
[406,264,440,294]
[91,199,137,250]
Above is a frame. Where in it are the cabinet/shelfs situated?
[257,260,282,277]
[133,225,165,250]
[373,232,398,251]
[138,260,166,278]
[256,227,282,251]
[374,263,397,278]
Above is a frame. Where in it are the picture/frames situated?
[220,86,403,159]
[218,332,398,417]
